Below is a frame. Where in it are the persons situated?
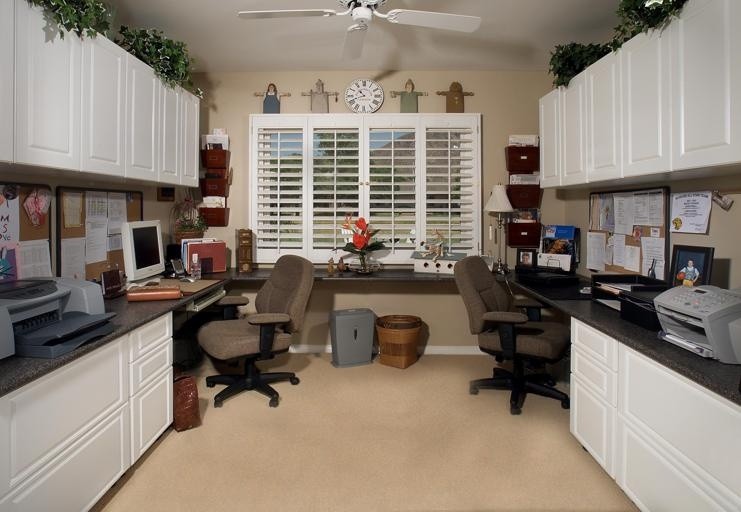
[520,254,530,264]
[676,259,699,285]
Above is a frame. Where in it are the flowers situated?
[343,216,386,259]
[168,194,209,234]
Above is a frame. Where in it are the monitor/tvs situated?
[121,220,165,288]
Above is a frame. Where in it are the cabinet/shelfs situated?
[0,306,178,509]
[197,147,234,228]
[505,142,543,250]
[538,0,741,195]
[0,0,203,189]
[569,310,741,510]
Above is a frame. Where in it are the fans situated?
[234,1,484,38]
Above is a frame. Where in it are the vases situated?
[348,253,383,275]
[173,229,206,247]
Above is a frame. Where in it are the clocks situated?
[342,77,386,115]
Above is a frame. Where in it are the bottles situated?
[338,257,346,273]
[191,251,202,278]
[328,257,335,276]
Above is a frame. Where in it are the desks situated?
[509,265,621,315]
[118,275,227,318]
[202,267,505,285]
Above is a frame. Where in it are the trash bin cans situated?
[330,308,374,368]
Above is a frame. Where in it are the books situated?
[126,287,181,302]
[537,225,577,271]
[508,205,540,223]
[181,238,226,273]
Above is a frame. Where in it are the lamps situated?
[485,181,516,276]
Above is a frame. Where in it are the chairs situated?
[454,253,578,418]
[199,253,316,410]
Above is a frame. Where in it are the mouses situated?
[179,277,194,282]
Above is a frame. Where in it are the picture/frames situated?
[517,247,539,267]
[668,241,718,294]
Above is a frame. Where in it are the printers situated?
[654,285,741,365]
[0,277,118,361]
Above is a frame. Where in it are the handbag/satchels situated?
[126,285,181,301]
